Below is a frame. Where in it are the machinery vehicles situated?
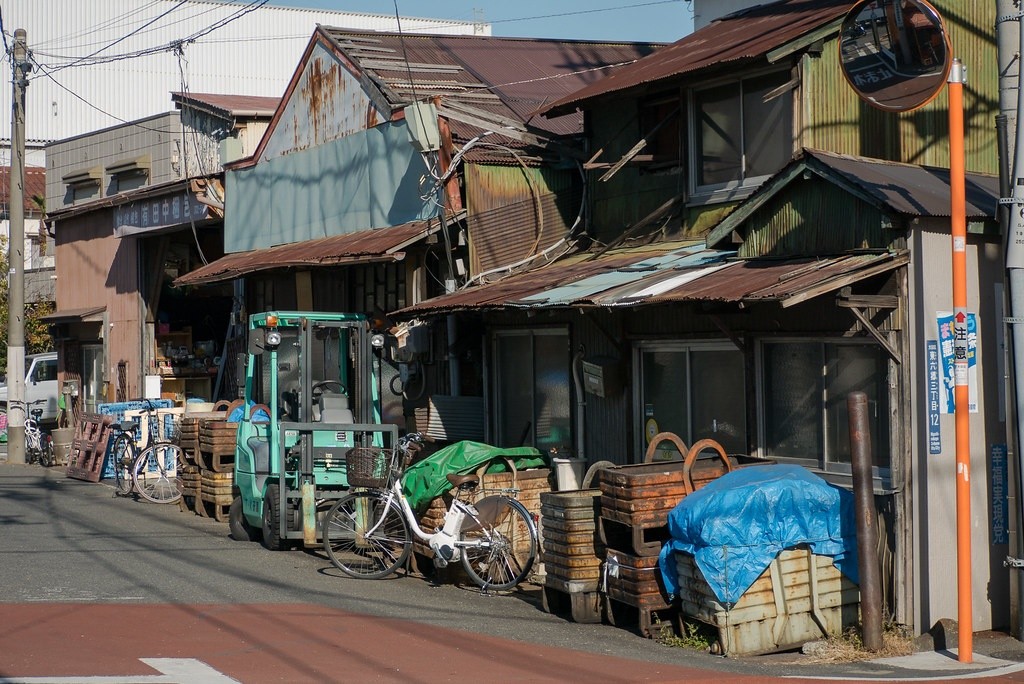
[230,310,401,553]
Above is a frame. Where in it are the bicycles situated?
[321,432,539,596]
[109,398,185,505]
[9,398,54,466]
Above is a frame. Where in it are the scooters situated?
[848,24,866,37]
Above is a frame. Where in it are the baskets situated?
[344,447,392,488]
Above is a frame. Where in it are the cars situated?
[0,352,57,426]
